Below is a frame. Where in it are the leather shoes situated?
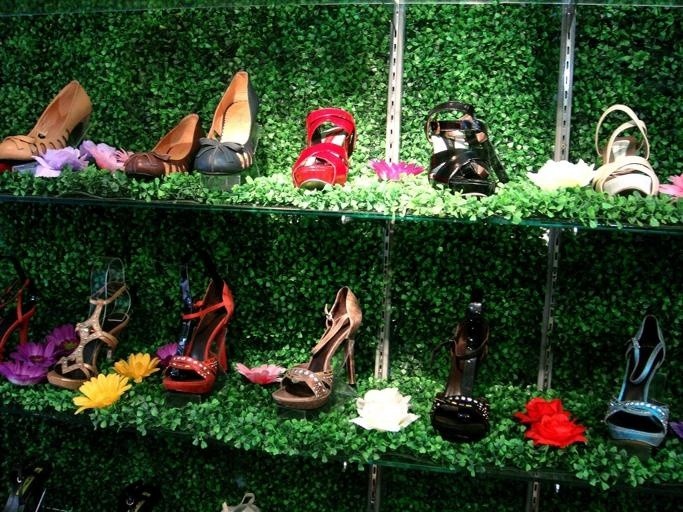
[123,113,201,180]
[194,70,260,177]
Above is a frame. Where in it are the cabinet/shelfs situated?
[1,2,683,512]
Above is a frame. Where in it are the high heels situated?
[270,286,364,409]
[162,277,235,397]
[1,79,95,164]
[1,275,36,353]
[47,281,131,391]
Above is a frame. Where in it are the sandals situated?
[292,107,359,188]
[601,313,671,449]
[425,100,509,197]
[593,103,660,199]
[430,282,491,443]
